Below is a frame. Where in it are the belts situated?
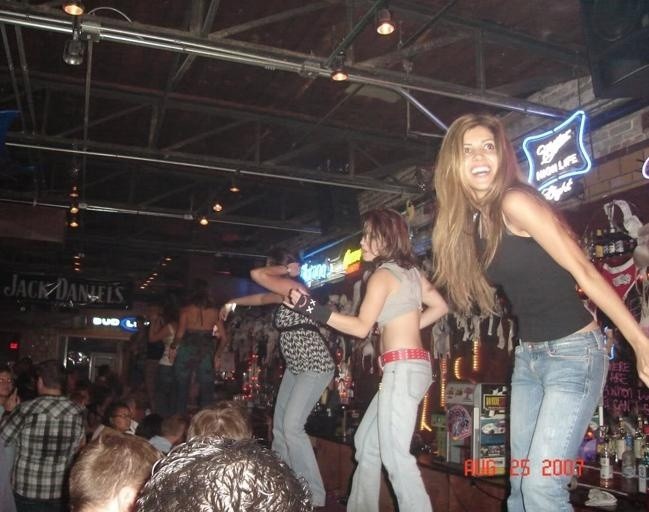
[379,349,429,367]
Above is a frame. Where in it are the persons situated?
[427,112,649,512]
[281,207,451,512]
[214,245,347,512]
[1,278,321,512]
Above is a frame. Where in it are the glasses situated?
[109,413,131,419]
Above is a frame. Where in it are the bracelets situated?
[284,260,291,276]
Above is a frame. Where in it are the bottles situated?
[598,435,614,487]
[637,445,648,496]
[620,442,635,479]
[635,417,646,460]
[615,417,627,464]
[578,225,636,260]
